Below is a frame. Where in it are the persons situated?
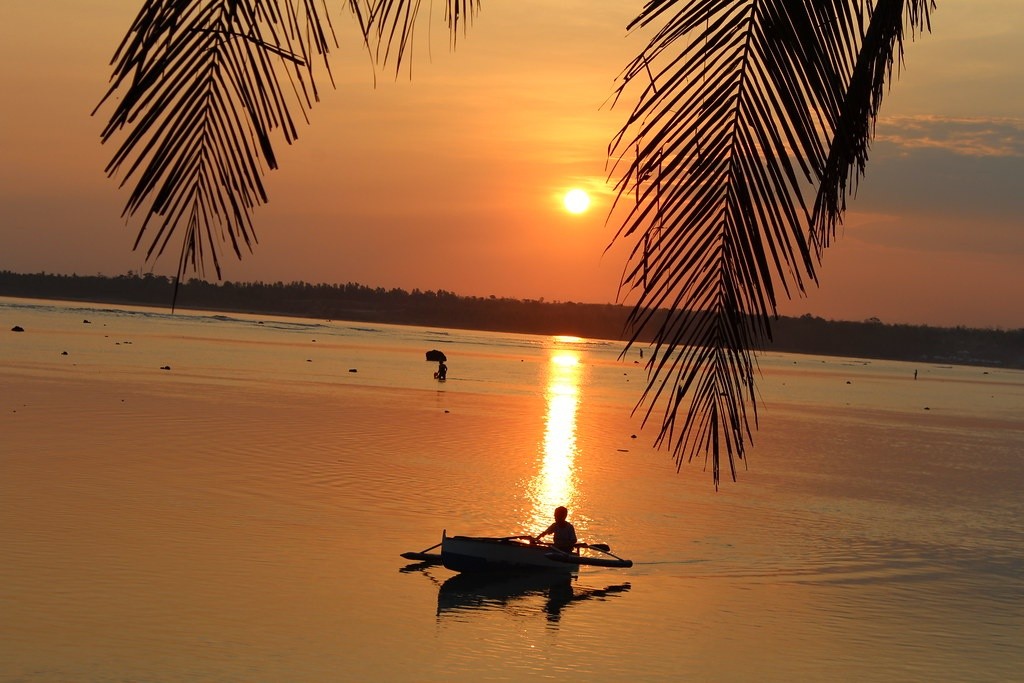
[640,348,643,358]
[914,369,917,380]
[530,506,577,553]
[438,361,447,380]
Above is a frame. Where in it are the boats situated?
[399,529,634,580]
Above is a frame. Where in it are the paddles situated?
[419,534,610,552]
